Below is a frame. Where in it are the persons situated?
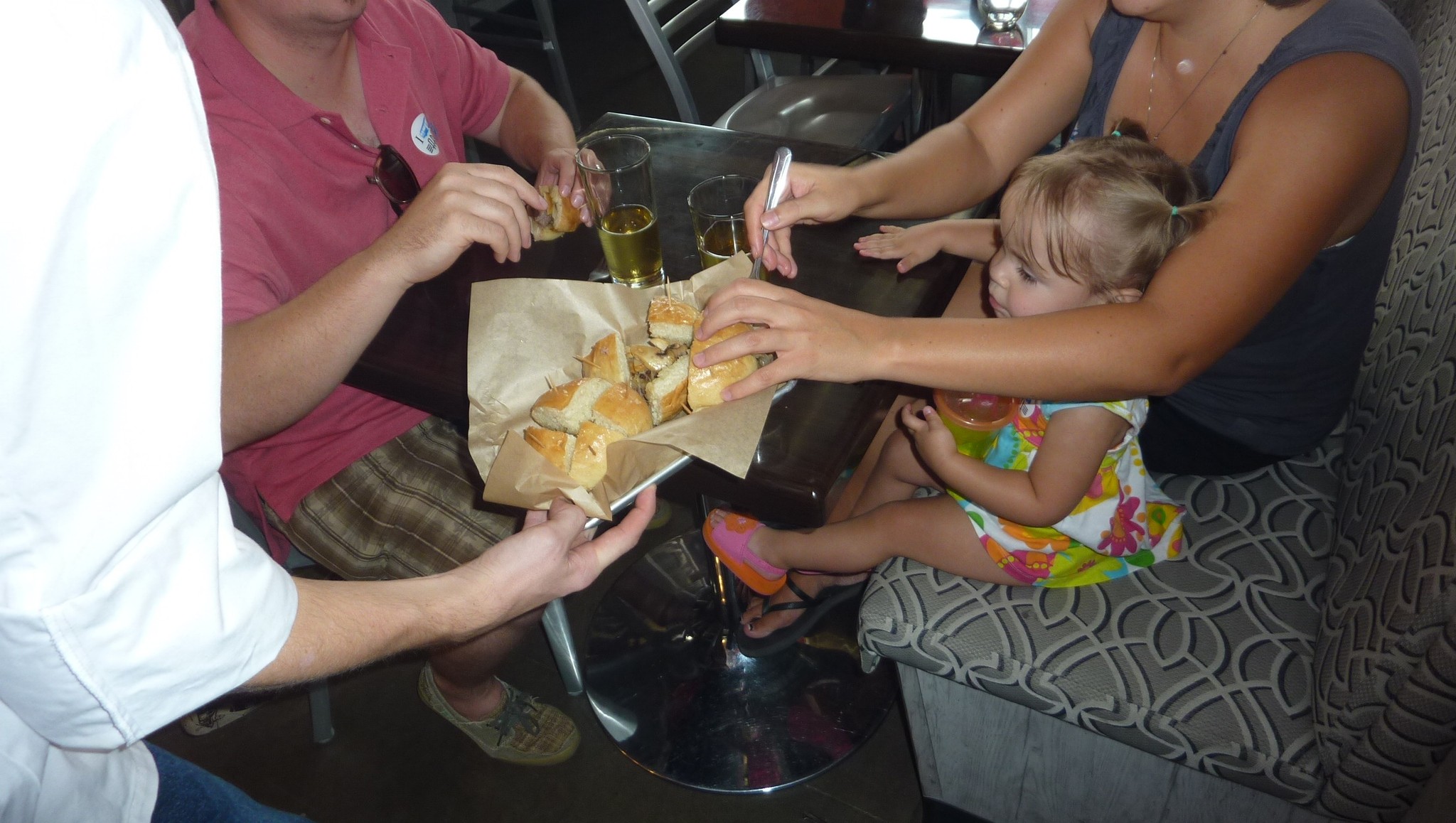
[702,116,1192,602]
[3,0,659,823]
[177,0,615,767]
[740,1,1426,661]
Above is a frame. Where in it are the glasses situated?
[366,145,422,217]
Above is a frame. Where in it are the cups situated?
[932,388,1019,463]
[687,173,768,282]
[977,0,1029,32]
[574,134,665,290]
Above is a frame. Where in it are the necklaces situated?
[1142,3,1268,143]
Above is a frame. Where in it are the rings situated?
[594,164,602,170]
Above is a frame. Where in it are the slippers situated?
[734,576,865,657]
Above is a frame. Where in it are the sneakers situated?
[417,661,580,765]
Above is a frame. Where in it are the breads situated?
[522,298,759,491]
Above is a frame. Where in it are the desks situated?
[718,0,1055,145]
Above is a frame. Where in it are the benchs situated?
[855,0,1456,823]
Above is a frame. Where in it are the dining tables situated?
[345,112,991,795]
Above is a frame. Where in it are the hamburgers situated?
[527,183,582,242]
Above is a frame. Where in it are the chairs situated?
[625,0,913,154]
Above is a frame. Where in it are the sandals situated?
[703,509,787,596]
[795,570,825,575]
